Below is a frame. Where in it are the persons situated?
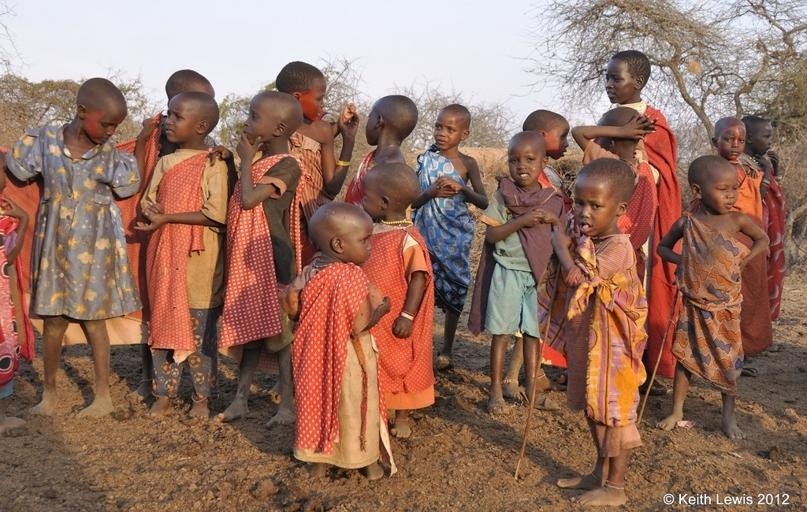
[1,51,785,508]
